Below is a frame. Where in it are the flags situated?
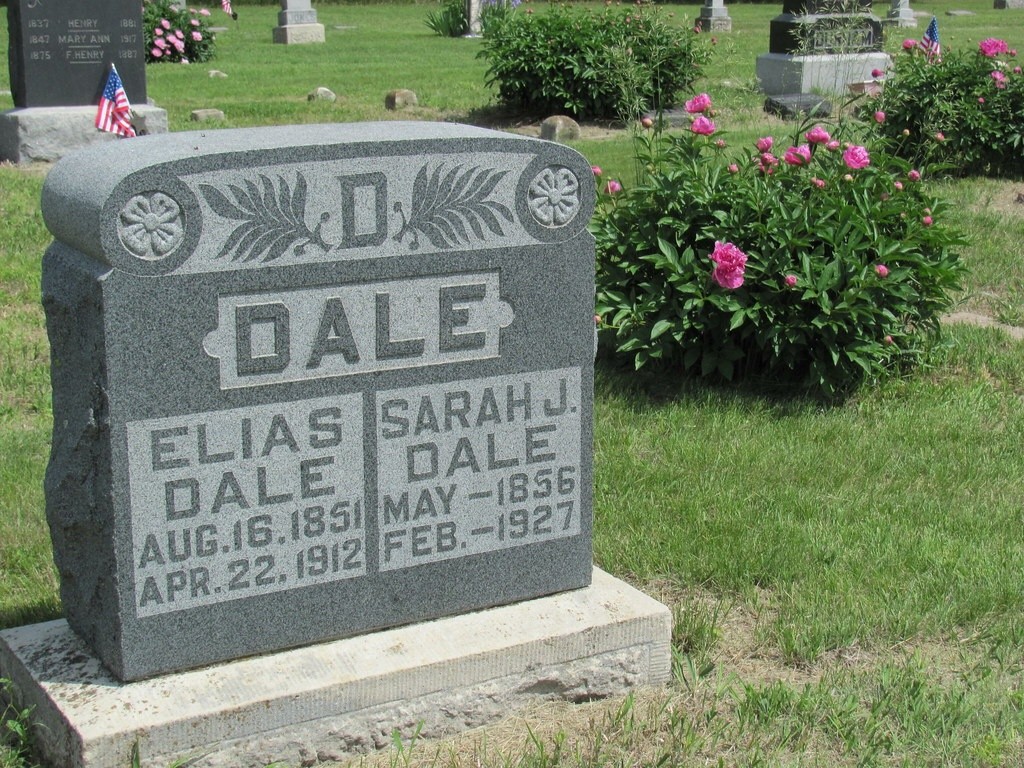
[95,69,137,137]
[919,14,940,65]
[222,0,233,16]
